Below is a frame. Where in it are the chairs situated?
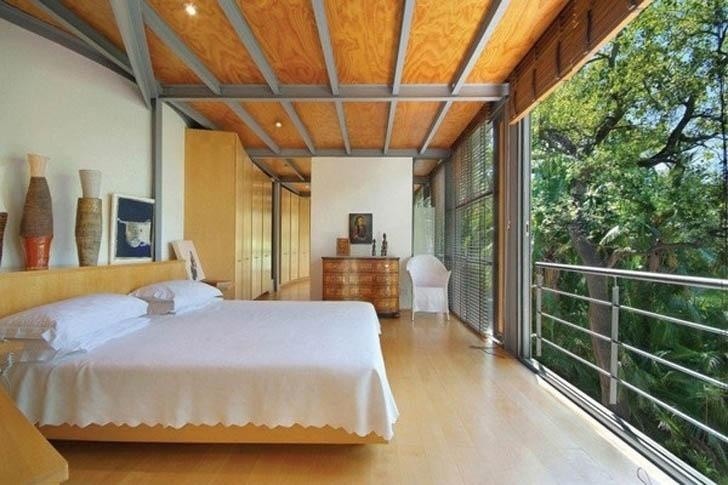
[405,252,453,322]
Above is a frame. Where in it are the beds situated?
[1,259,398,448]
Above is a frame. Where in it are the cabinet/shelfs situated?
[321,254,400,320]
[186,129,310,300]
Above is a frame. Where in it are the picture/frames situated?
[348,211,373,245]
[109,194,156,266]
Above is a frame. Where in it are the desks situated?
[0,380,69,484]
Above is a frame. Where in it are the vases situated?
[74,167,103,268]
[0,193,9,264]
[16,150,54,270]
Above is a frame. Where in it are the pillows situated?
[130,277,223,313]
[0,293,149,361]
